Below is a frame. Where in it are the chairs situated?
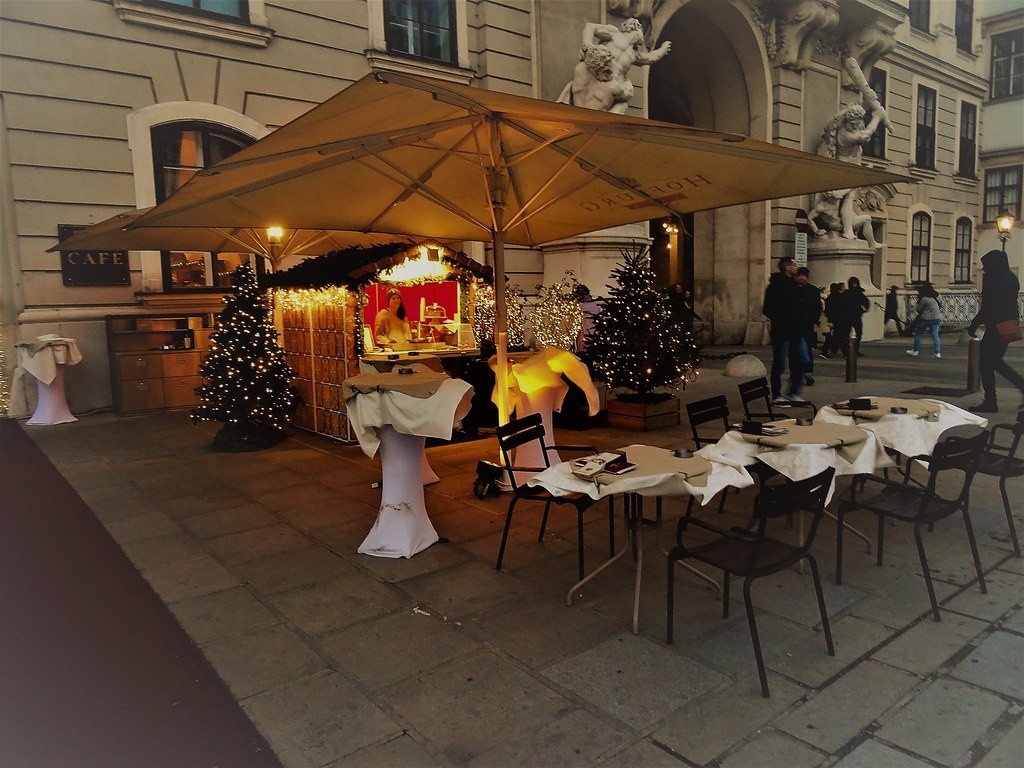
[665,464,836,699]
[927,409,1024,560]
[685,394,765,531]
[494,412,617,582]
[738,375,817,423]
[832,429,990,623]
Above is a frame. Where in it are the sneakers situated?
[932,352,942,359]
[771,396,792,408]
[783,393,807,402]
[906,348,920,356]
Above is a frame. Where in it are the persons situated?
[787,266,823,385]
[573,284,602,351]
[837,89,886,240]
[968,250,1024,413]
[572,44,633,112]
[374,288,412,344]
[807,191,887,249]
[886,280,942,359]
[582,18,672,77]
[818,277,870,361]
[762,257,812,408]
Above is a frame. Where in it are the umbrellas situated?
[45,205,465,275]
[120,66,922,490]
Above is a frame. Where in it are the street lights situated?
[995,207,1015,252]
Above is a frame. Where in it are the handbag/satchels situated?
[994,317,1024,345]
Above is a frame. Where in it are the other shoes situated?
[787,375,793,382]
[805,373,815,386]
[819,352,831,360]
[842,354,848,361]
[969,398,998,414]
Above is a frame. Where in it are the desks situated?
[358,353,445,485]
[343,371,475,559]
[488,346,600,495]
[13,333,83,426]
[734,416,873,577]
[527,444,755,636]
[824,395,988,499]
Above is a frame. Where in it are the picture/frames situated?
[363,324,375,349]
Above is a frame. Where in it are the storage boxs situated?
[849,398,871,410]
[604,449,627,467]
[742,420,763,436]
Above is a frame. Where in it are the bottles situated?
[183,334,191,349]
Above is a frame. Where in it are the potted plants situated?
[576,238,703,433]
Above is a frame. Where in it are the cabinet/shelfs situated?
[105,312,220,417]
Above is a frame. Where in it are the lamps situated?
[471,458,505,501]
